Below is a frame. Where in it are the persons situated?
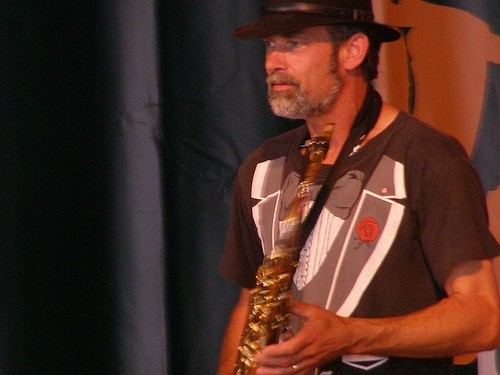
[218,0,500,375]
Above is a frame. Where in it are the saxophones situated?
[218,121,335,375]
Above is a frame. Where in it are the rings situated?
[293,364,300,374]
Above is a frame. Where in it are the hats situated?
[234,0,401,42]
